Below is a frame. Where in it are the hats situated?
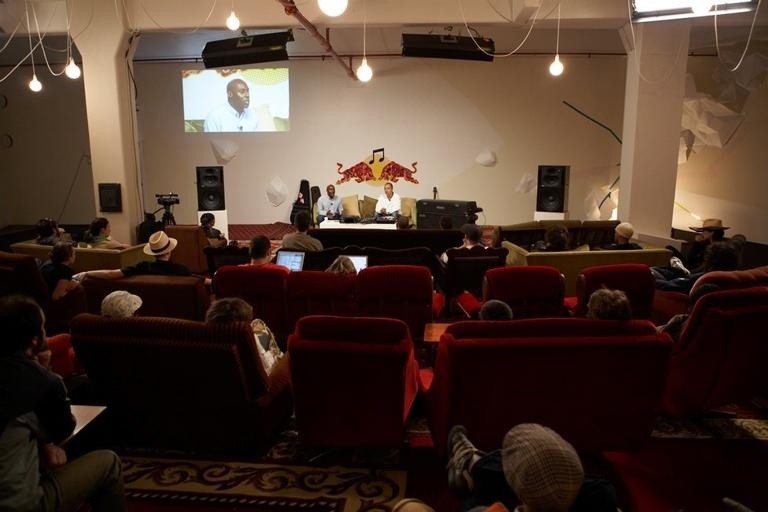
[501,422,584,512]
[615,223,634,239]
[100,290,142,318]
[143,231,177,256]
[689,219,730,231]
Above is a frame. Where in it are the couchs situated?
[313,194,417,229]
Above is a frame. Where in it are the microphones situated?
[432,186,437,199]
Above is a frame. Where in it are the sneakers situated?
[447,424,478,491]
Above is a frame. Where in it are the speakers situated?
[537,164,570,212]
[196,165,225,211]
[581,220,622,244]
[542,220,581,251]
[498,222,546,251]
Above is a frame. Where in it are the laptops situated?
[274,252,304,272]
[339,256,368,275]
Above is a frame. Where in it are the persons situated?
[392,423,586,512]
[204,294,285,379]
[477,300,513,321]
[0,296,125,512]
[32,216,213,318]
[315,185,346,224]
[397,211,492,266]
[238,213,355,277]
[525,219,746,337]
[204,80,274,132]
[374,182,401,221]
[201,213,228,256]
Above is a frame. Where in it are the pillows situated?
[362,195,378,217]
[401,200,410,217]
[339,195,362,221]
